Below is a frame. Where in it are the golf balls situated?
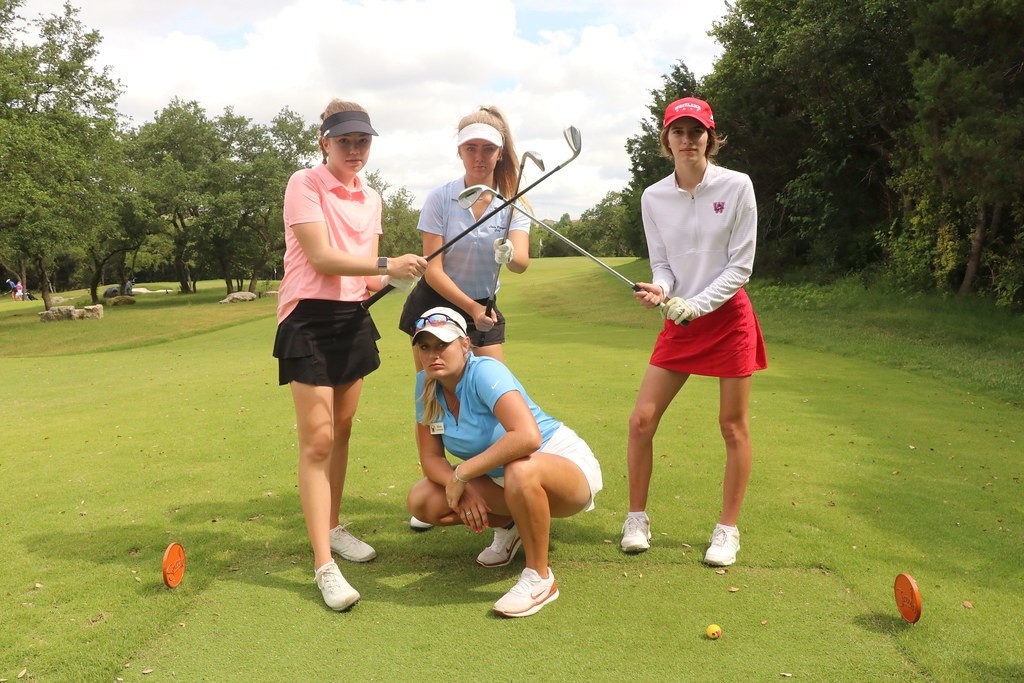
[707,624,722,639]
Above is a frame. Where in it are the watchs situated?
[377,257,389,275]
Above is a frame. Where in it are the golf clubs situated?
[360,125,583,312]
[476,150,546,348]
[456,184,688,328]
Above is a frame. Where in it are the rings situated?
[448,502,452,505]
[466,512,471,516]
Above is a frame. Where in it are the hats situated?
[320,110,378,137]
[456,122,503,147]
[662,97,716,130]
[412,306,467,342]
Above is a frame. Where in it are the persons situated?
[274,99,427,610]
[407,306,602,617]
[6,279,23,301]
[621,98,767,566]
[399,105,530,529]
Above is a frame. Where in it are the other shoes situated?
[408,515,434,529]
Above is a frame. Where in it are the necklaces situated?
[479,193,489,199]
[678,177,703,199]
[445,397,458,413]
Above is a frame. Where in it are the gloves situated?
[381,274,421,294]
[493,237,514,264]
[661,296,699,326]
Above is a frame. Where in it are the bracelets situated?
[453,465,467,482]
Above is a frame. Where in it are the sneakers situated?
[330,524,377,563]
[314,557,360,614]
[703,523,741,567]
[621,512,652,553]
[492,566,560,619]
[476,520,522,568]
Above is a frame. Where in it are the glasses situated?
[414,312,467,337]
